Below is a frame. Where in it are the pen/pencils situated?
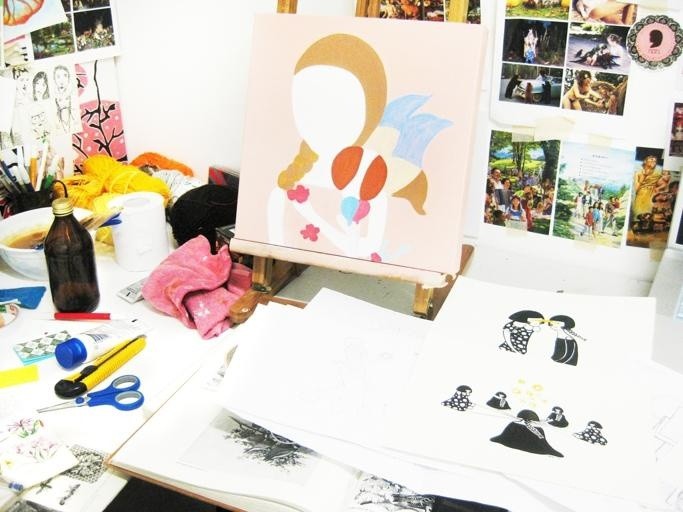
[0,143,59,206]
[54,310,111,320]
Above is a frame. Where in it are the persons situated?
[483,151,682,243]
[9,65,32,142]
[29,71,57,144]
[502,1,638,116]
[50,65,79,138]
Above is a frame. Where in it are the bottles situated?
[44,197,101,313]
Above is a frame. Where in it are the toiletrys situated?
[54,323,127,368]
[116,278,149,304]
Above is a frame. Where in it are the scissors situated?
[37,375,145,413]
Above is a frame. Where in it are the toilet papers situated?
[109,191,166,271]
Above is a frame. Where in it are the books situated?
[105,365,437,511]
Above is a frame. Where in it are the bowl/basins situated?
[0,206,96,284]
